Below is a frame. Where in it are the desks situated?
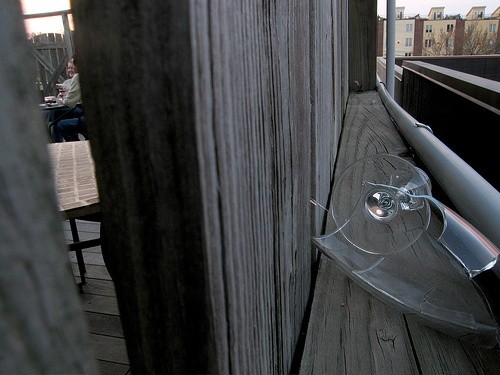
[39,103,83,144]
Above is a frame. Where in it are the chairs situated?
[48,102,82,143]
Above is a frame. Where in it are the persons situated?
[56,53,89,142]
[56,58,76,99]
